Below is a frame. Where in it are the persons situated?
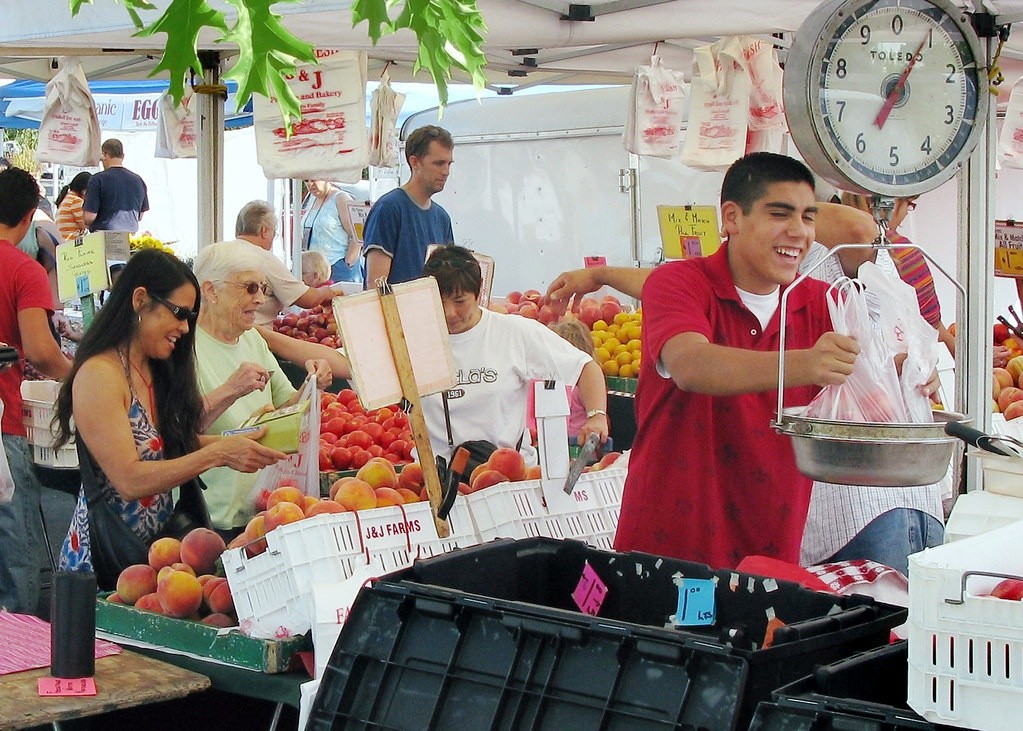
[172,239,332,540]
[544,201,945,578]
[235,200,343,330]
[0,167,73,616]
[551,319,594,446]
[361,125,455,290]
[55,172,92,241]
[53,247,290,574]
[259,246,608,463]
[612,151,940,571]
[301,179,363,283]
[841,192,1010,368]
[82,139,149,234]
[302,250,335,287]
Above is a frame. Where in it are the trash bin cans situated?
[748,639,1010,731]
[40,168,64,196]
[303,535,908,731]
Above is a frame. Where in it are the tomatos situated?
[319,390,417,471]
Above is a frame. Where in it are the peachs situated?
[487,290,622,330]
[991,355,1023,421]
[102,450,618,631]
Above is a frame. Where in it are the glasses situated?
[904,199,918,211]
[149,292,196,323]
[424,259,480,270]
[213,279,270,295]
[304,179,315,182]
[411,126,442,155]
[273,231,279,240]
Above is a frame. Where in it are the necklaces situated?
[124,353,155,422]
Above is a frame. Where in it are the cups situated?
[50,570,96,679]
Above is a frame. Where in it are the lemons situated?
[585,306,642,379]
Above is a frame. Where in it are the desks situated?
[0,613,211,731]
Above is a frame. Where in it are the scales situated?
[771,0,993,486]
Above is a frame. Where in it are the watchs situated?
[587,409,606,419]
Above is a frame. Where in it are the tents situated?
[0,0,1023,493]
[0,80,291,269]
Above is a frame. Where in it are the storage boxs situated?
[20,339,1020,731]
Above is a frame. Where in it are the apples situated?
[991,324,1023,368]
[272,304,342,348]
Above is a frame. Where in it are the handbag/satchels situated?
[800,260,941,423]
[447,441,498,483]
[82,454,214,593]
